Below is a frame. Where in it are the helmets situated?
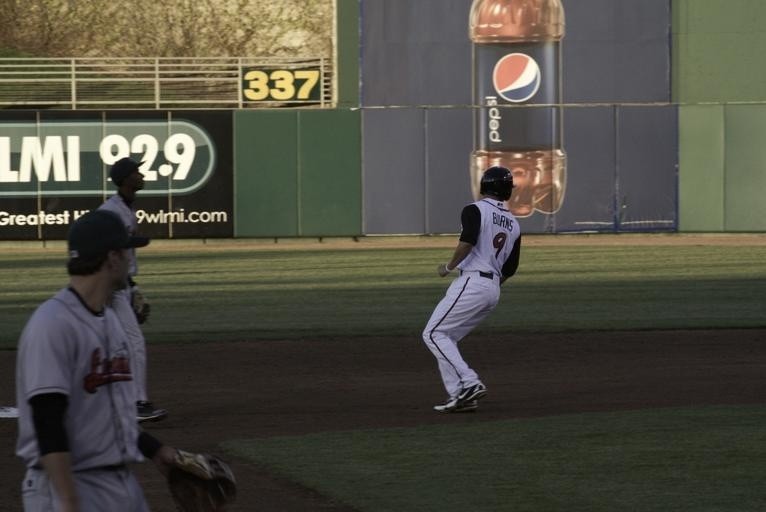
[480,167,514,201]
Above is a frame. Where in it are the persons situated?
[421,167,522,413]
[94,158,168,423]
[12,209,236,511]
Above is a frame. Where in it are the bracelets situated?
[445,264,452,274]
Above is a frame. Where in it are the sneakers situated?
[433,395,479,413]
[134,400,169,424]
[446,382,488,408]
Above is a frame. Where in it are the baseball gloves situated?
[165,448,237,511]
[132,290,150,324]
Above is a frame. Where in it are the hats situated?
[110,157,144,183]
[67,209,150,265]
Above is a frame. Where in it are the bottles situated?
[469,0,567,218]
